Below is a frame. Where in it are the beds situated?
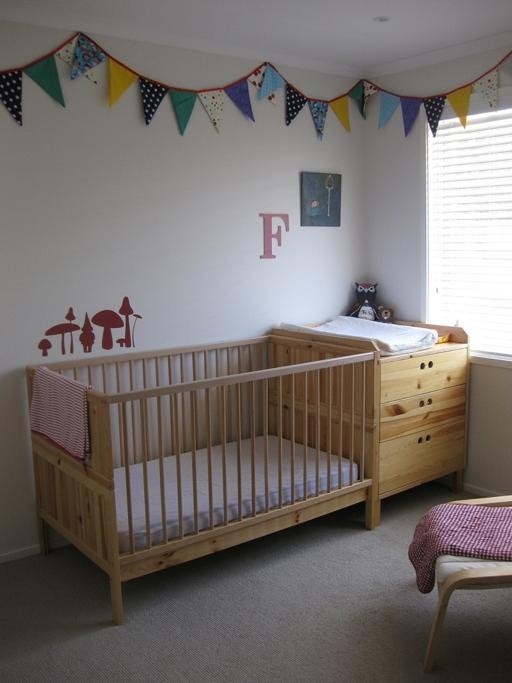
[17,334,382,618]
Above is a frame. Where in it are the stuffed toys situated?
[376,303,394,323]
[349,279,386,321]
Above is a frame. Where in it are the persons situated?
[306,198,320,216]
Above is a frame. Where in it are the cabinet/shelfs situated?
[275,320,468,531]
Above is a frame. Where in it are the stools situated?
[406,485,512,673]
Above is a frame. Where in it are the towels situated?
[27,365,93,461]
[311,316,436,351]
[409,499,511,592]
